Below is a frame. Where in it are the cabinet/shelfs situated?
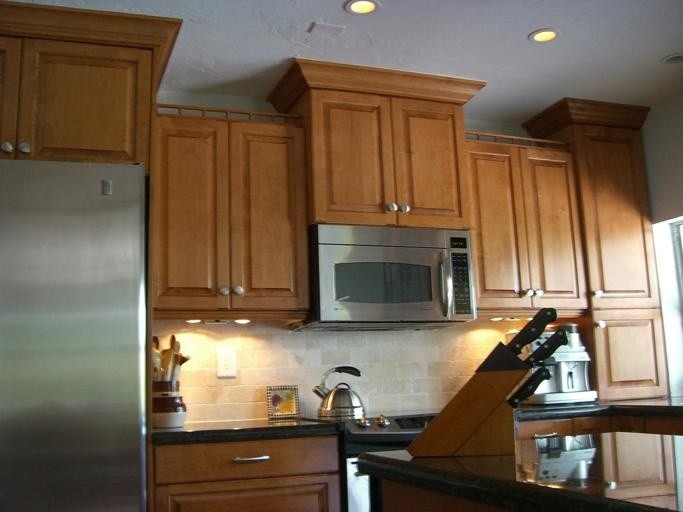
[148,104,310,321]
[357,432,682,512]
[0,1,184,175]
[521,97,660,309]
[597,434,676,499]
[147,417,348,512]
[265,58,487,231]
[463,132,589,318]
[579,308,669,402]
[611,398,683,435]
[515,399,611,440]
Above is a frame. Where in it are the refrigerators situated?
[1,160,148,512]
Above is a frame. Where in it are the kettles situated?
[311,364,367,420]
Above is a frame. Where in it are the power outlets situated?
[216,346,236,378]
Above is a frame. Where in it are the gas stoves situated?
[341,413,436,456]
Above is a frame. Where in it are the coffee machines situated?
[522,322,598,404]
[531,432,597,490]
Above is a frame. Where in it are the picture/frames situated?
[266,385,300,419]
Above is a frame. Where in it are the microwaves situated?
[290,224,477,332]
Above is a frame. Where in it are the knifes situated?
[481,304,569,407]
